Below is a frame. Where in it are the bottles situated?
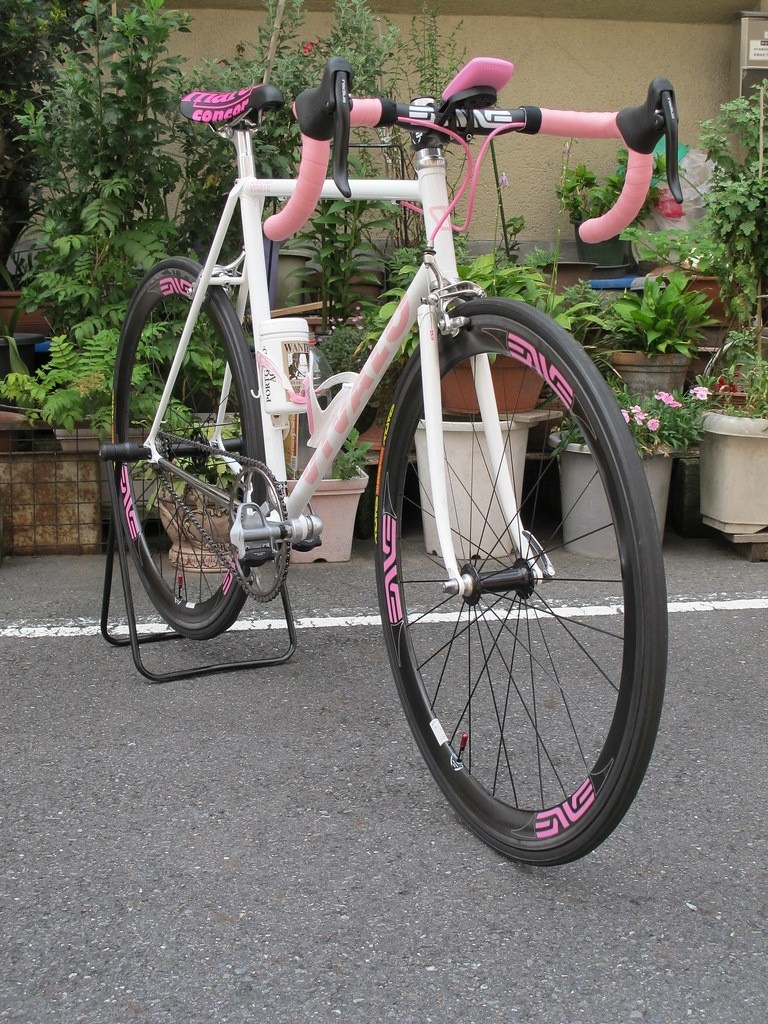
[284,332,333,480]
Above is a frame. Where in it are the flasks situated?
[258,317,309,414]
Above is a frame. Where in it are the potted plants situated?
[0,0,768,572]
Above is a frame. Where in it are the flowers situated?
[310,305,375,375]
[696,325,768,419]
[550,373,713,459]
[488,138,581,312]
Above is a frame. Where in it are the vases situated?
[699,410,768,535]
[548,432,673,561]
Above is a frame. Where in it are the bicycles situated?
[94,57,687,869]
